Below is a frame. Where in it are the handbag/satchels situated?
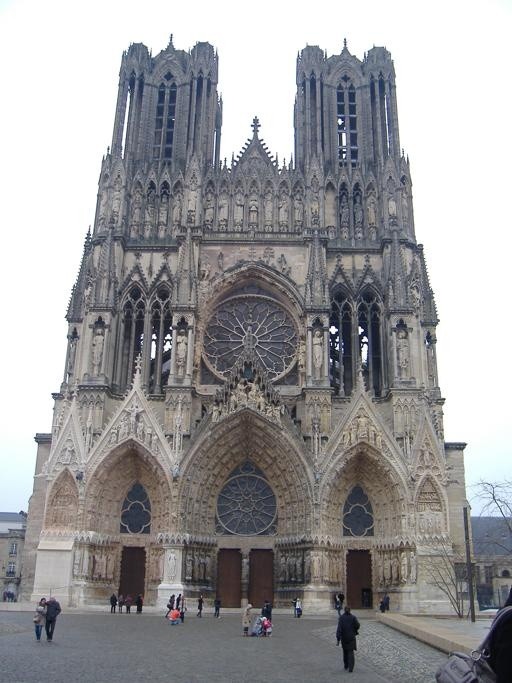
[33,616,40,622]
[435,650,497,682]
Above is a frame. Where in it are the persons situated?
[2,589,14,603]
[36,594,62,642]
[254,599,274,638]
[110,592,117,613]
[334,591,345,611]
[379,591,390,613]
[213,597,221,618]
[125,593,133,613]
[134,591,144,614]
[33,597,47,642]
[290,596,303,618]
[362,590,371,609]
[335,605,361,673]
[164,593,188,625]
[241,603,253,637]
[480,584,511,683]
[117,594,125,614]
[196,594,204,618]
